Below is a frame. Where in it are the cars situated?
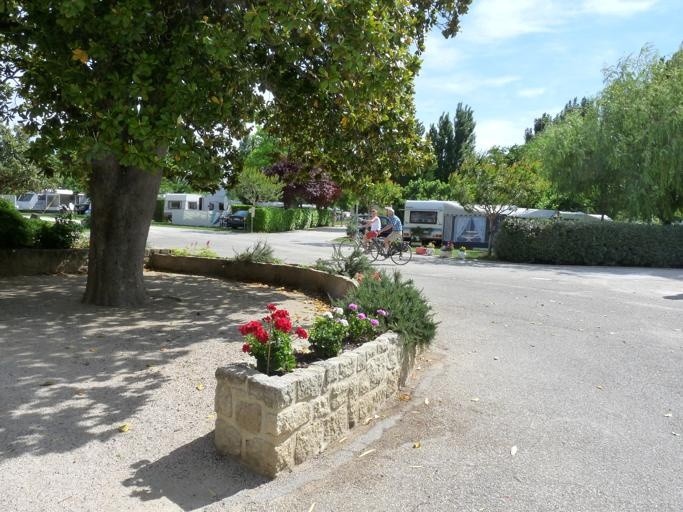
[225,210,249,230]
[345,214,392,237]
[75,203,89,215]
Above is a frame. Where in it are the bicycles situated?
[357,231,413,266]
[337,227,388,261]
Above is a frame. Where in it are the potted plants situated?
[410,225,466,258]
[346,212,358,240]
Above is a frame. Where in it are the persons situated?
[375,207,402,258]
[360,209,381,255]
